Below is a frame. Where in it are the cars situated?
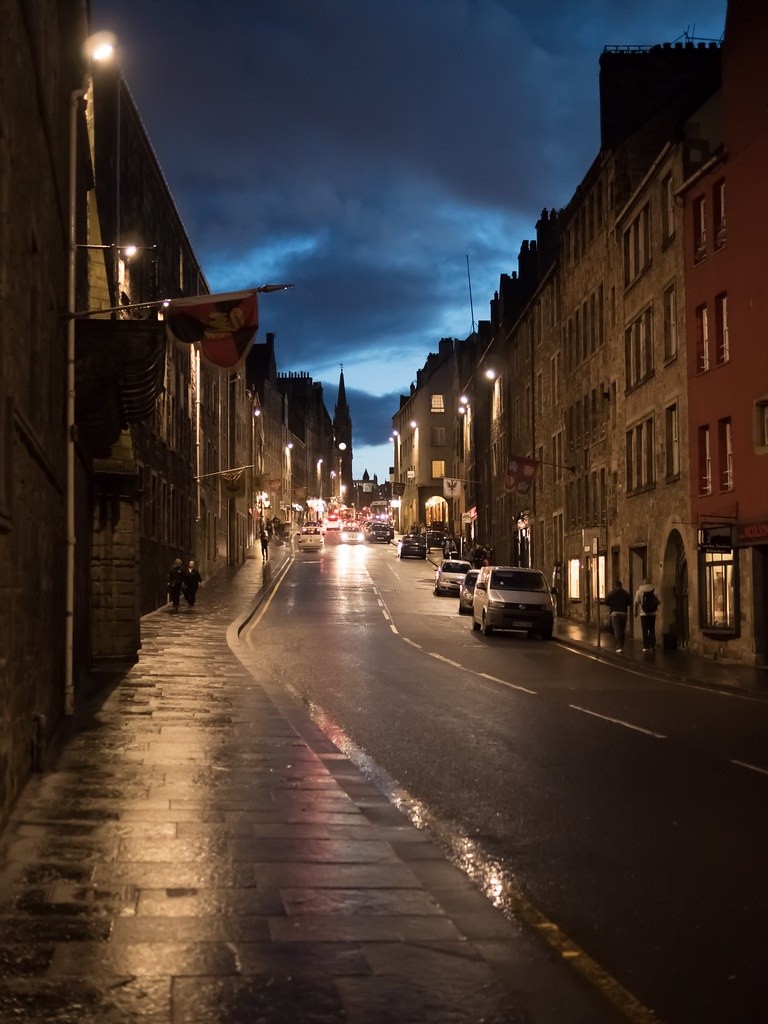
[427,530,445,549]
[398,534,428,560]
[457,568,482,615]
[296,517,395,552]
[432,559,474,598]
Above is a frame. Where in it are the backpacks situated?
[640,588,657,613]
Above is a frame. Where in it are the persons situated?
[165,559,183,614]
[604,580,634,653]
[440,537,457,559]
[180,560,202,608]
[258,516,264,534]
[260,531,269,565]
[265,519,273,541]
[634,578,661,652]
[472,544,487,569]
[270,515,281,529]
[409,522,428,535]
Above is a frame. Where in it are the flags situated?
[393,482,405,496]
[269,479,282,492]
[329,497,338,505]
[159,289,258,377]
[443,478,462,497]
[294,488,308,500]
[502,456,539,498]
[220,468,245,484]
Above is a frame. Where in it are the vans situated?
[472,566,559,642]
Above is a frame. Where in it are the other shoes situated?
[651,647,656,653]
[643,649,649,654]
[616,648,622,653]
